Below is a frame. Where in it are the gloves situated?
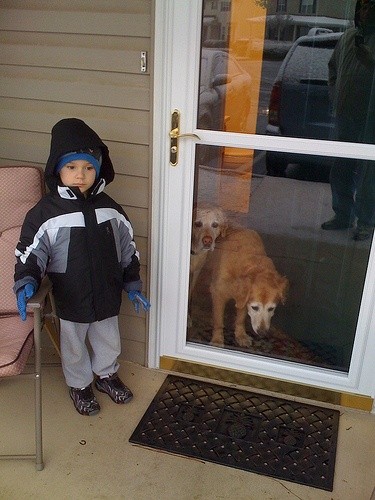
[16,283,35,321]
[128,290,151,314]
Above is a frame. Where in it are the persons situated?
[13,118,151,415]
[320,0,375,241]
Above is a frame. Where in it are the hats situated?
[55,140,102,178]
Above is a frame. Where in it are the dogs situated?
[200,211,290,350]
[187,200,240,329]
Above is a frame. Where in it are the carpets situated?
[128,375,340,492]
[203,324,341,370]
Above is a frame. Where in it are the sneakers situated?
[94,372,134,404]
[69,383,100,416]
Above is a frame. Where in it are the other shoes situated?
[353,222,374,241]
[321,215,353,230]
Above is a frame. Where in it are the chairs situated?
[0,165,61,472]
